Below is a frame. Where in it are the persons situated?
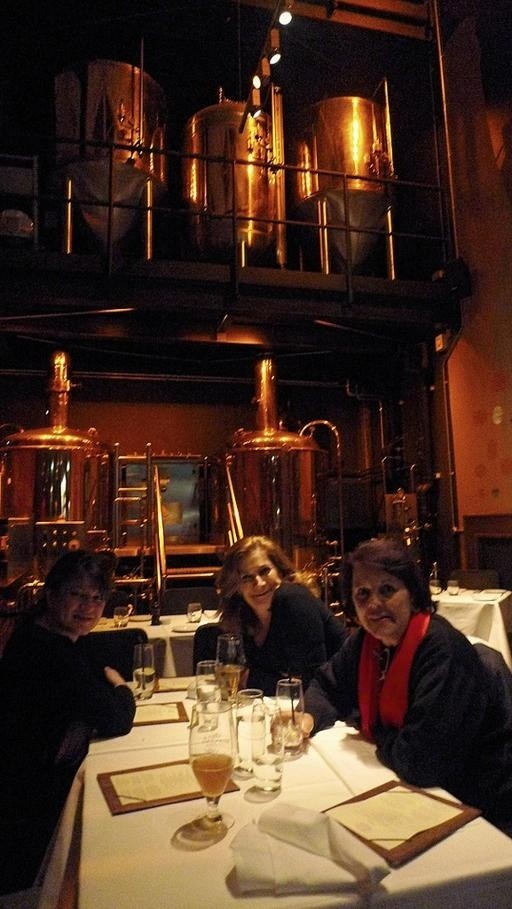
[268,537,511,832]
[0,548,137,893]
[208,536,349,696]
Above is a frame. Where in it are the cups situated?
[131,633,304,834]
[114,606,129,628]
[430,580,441,595]
[187,603,202,622]
[448,581,459,595]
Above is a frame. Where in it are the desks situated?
[72,609,227,676]
[428,586,510,666]
[77,674,511,907]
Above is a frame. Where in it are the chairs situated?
[448,568,500,590]
[193,622,231,671]
[75,628,151,680]
